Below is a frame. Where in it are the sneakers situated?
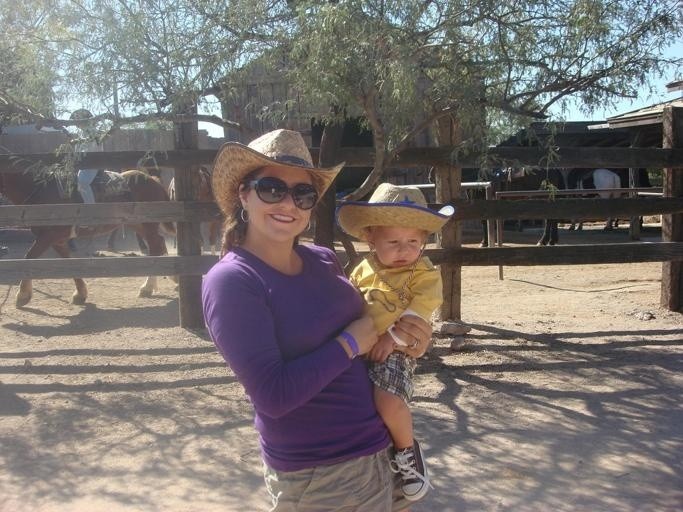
[389,438,434,501]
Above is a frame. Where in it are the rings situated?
[410,341,417,349]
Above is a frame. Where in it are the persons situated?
[142,150,162,177]
[334,180,453,500]
[197,130,408,511]
[65,110,98,217]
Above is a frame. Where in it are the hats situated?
[209,129,346,215]
[337,183,456,241]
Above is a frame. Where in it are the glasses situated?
[247,177,319,210]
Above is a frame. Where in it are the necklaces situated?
[247,242,297,268]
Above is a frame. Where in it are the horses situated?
[0,146,223,308]
[567,168,622,231]
[462,164,565,247]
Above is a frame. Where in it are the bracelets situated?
[339,330,359,362]
